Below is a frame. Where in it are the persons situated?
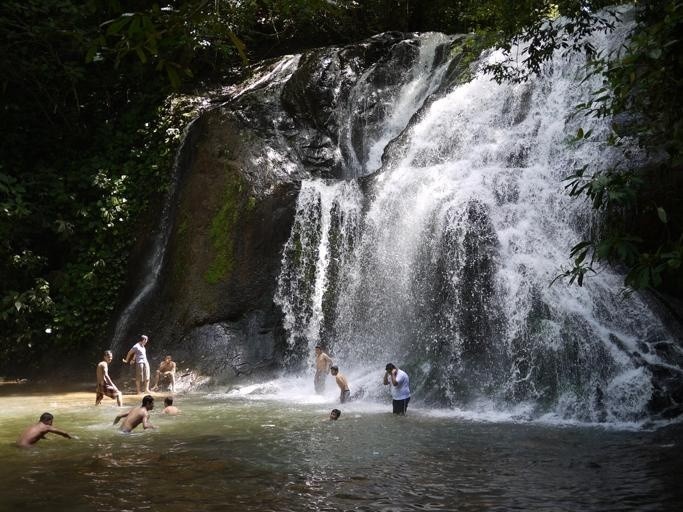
[159,396,180,415]
[313,346,333,394]
[112,394,159,432]
[329,408,341,421]
[95,350,122,406]
[150,355,177,393]
[15,412,72,448]
[384,363,411,416]
[331,365,350,403]
[122,334,152,394]
